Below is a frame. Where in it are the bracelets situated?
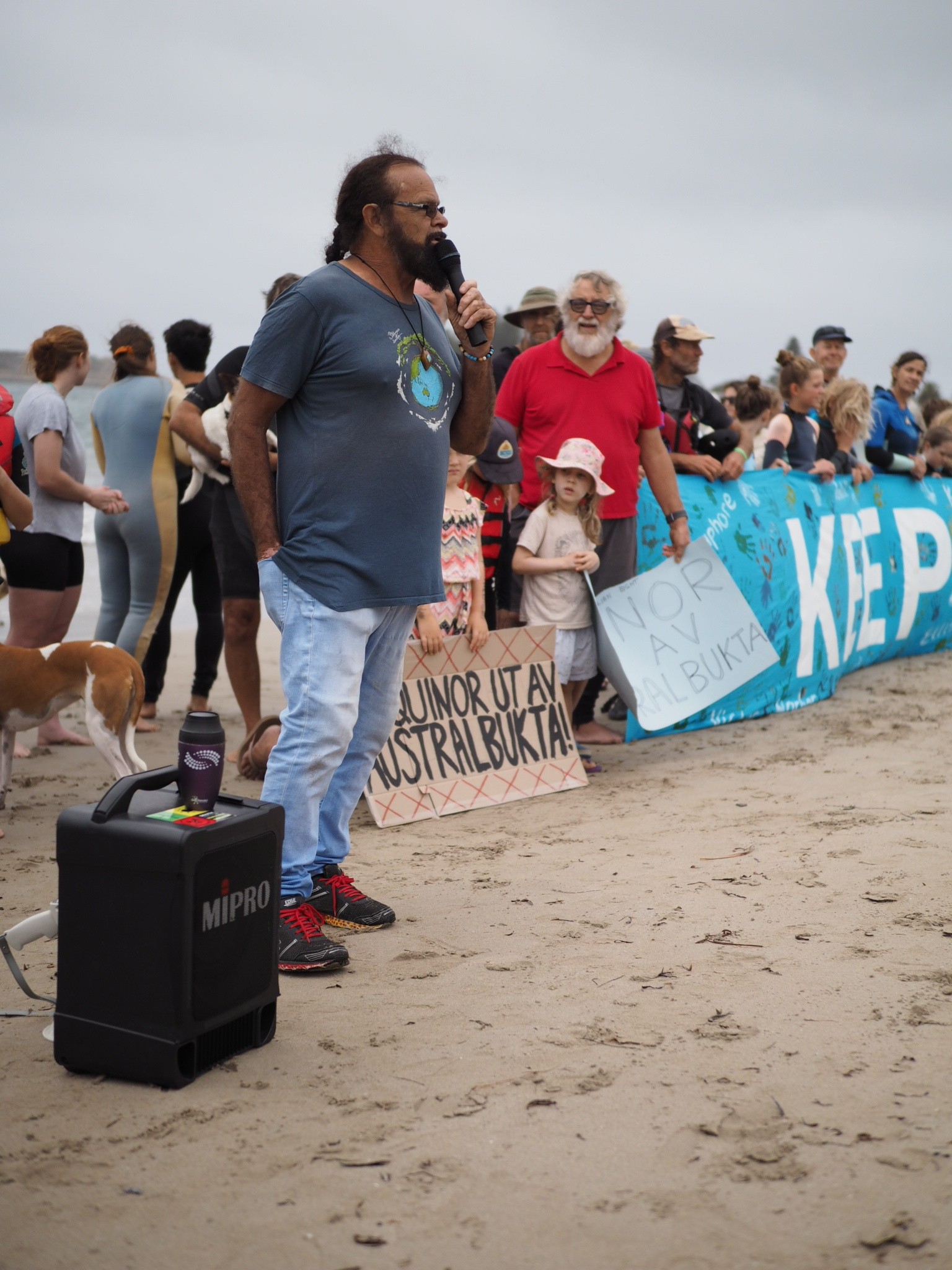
[258,545,278,561]
[734,447,749,462]
[664,510,688,525]
[459,343,494,362]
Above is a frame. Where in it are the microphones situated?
[433,239,488,347]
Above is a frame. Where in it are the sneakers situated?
[311,864,396,932]
[277,896,349,971]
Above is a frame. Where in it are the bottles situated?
[178,712,225,810]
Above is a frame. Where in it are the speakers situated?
[52,781,286,1091]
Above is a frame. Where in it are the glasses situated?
[385,200,445,218]
[569,298,610,314]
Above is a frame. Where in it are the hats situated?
[813,326,852,347]
[475,416,524,485]
[535,438,615,498]
[503,287,558,328]
[654,315,715,342]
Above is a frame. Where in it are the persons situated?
[407,287,952,631]
[227,153,495,977]
[512,438,615,774]
[0,325,129,759]
[495,272,690,745]
[409,445,489,657]
[91,272,303,781]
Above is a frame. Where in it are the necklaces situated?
[898,402,911,425]
[350,251,433,371]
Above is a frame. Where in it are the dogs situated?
[0,621,145,812]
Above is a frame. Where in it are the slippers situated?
[578,754,602,773]
[237,716,280,776]
